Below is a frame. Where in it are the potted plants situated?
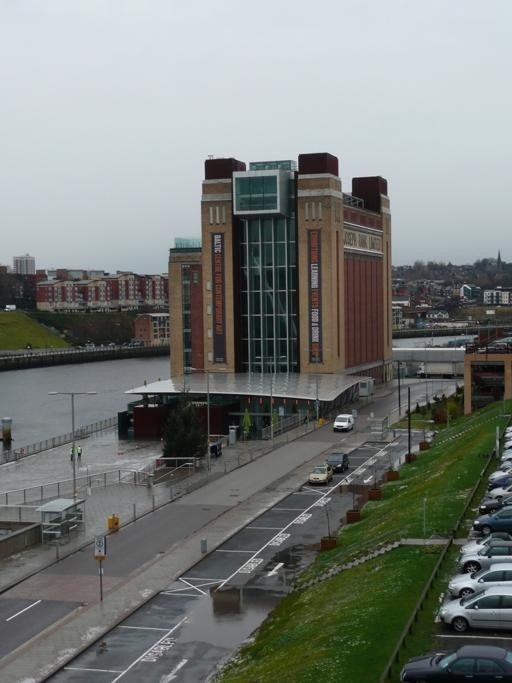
[405,452,416,463]
[419,427,429,450]
[321,508,338,549]
[368,463,381,499]
[384,461,399,481]
[346,485,360,522]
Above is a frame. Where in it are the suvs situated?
[308,462,334,484]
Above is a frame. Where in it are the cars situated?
[400,640,512,681]
[439,423,512,633]
[325,452,349,471]
[479,335,512,354]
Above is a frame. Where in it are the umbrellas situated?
[242,408,251,433]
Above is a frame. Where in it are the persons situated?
[70,447,73,461]
[76,445,82,462]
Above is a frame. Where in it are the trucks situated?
[416,360,455,378]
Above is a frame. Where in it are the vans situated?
[333,413,354,431]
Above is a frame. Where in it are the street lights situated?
[47,389,97,513]
[189,366,213,473]
